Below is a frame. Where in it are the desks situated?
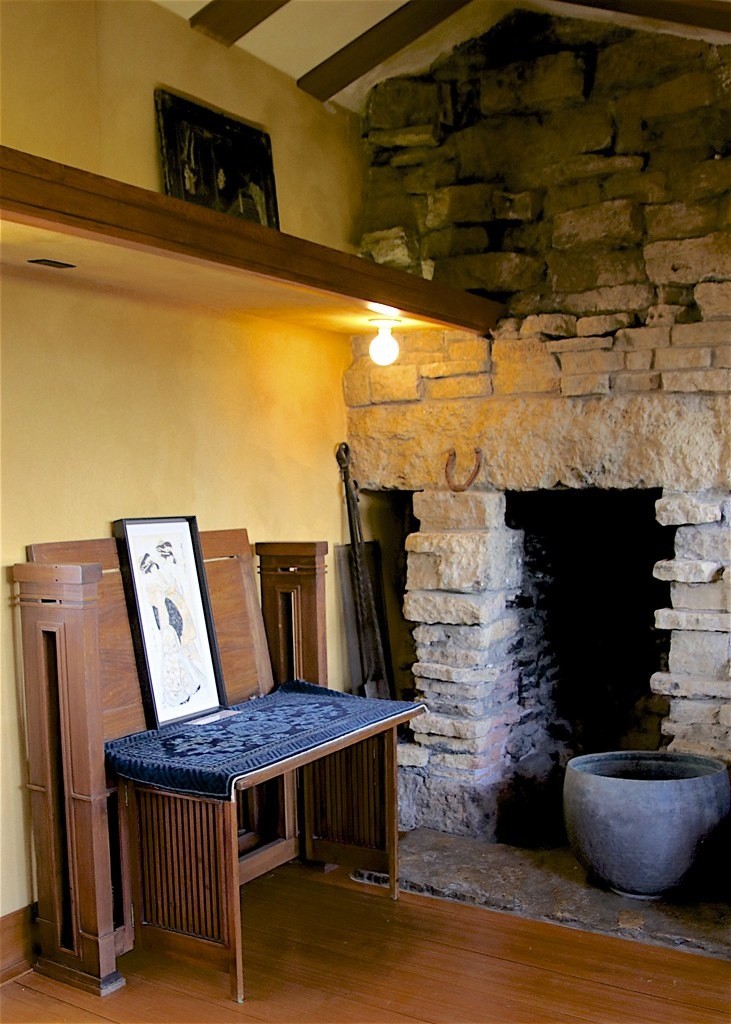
[104,679,427,1003]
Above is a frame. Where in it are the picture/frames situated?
[118,515,228,731]
[153,90,281,232]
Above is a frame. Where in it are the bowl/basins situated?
[563,749,731,902]
